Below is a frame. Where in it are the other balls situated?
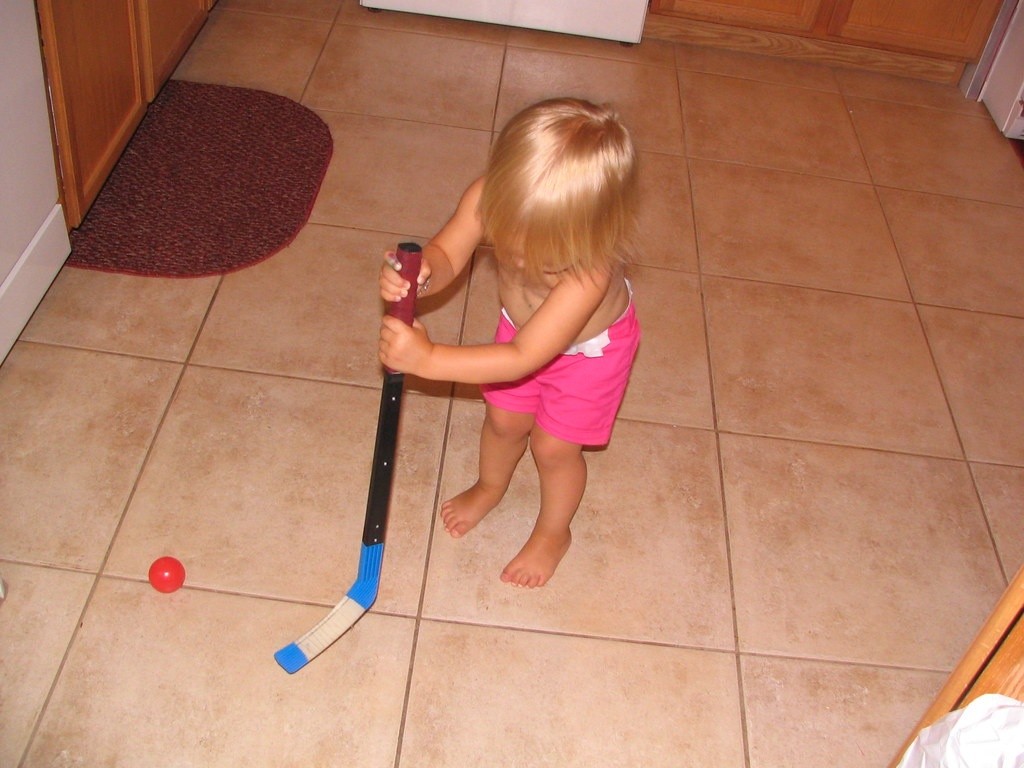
[148,556,187,594]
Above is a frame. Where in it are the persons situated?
[379,98,641,587]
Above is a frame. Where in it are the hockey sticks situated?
[273,243,424,675]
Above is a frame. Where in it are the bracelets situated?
[417,278,429,296]
